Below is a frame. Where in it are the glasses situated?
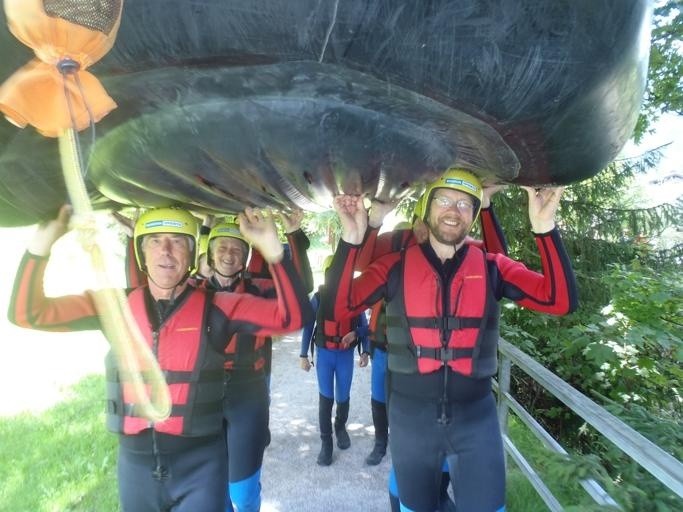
[432,197,474,211]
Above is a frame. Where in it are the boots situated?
[364,398,389,466]
[334,399,352,448]
[314,392,334,467]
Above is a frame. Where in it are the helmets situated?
[131,205,198,277]
[418,165,487,232]
[199,232,210,274]
[322,252,333,276]
[205,224,254,276]
[392,222,413,232]
[412,193,425,227]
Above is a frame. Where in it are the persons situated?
[113,204,315,511]
[8,203,317,512]
[357,181,509,465]
[362,300,389,465]
[300,267,372,465]
[326,168,578,512]
